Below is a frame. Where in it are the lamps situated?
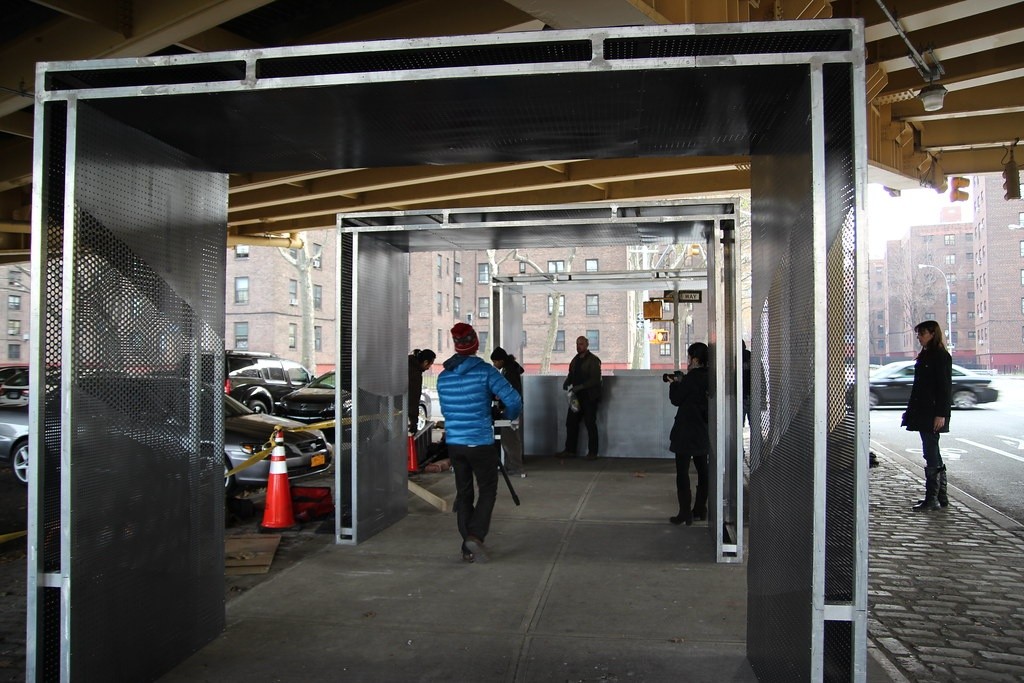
[918,78,948,111]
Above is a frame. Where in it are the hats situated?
[490,347,508,361]
[451,323,480,355]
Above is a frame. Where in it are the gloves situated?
[409,423,418,436]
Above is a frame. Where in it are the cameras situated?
[663,371,683,384]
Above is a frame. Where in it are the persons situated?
[668,342,710,525]
[408,349,436,436]
[558,336,602,459]
[435,322,522,564]
[490,347,524,475]
[741,340,766,441]
[900,321,955,511]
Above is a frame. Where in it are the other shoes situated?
[462,540,474,562]
[582,454,598,461]
[558,449,577,458]
[465,536,493,565]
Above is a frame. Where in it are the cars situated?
[169,352,350,433]
[0,371,332,502]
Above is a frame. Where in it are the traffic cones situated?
[259,428,305,535]
[407,425,421,476]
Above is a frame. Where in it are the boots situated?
[669,488,693,526]
[691,485,708,520]
[912,463,949,509]
